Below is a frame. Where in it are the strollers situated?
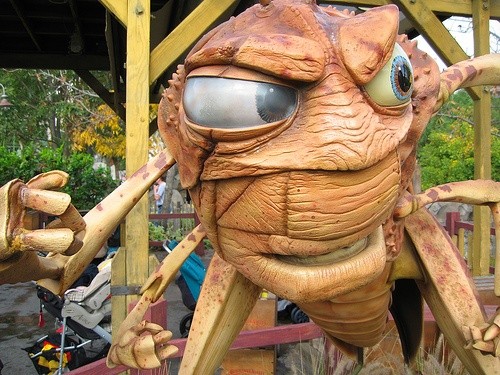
[26,252,113,375]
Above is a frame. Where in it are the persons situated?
[62,240,114,294]
[154,174,174,229]
